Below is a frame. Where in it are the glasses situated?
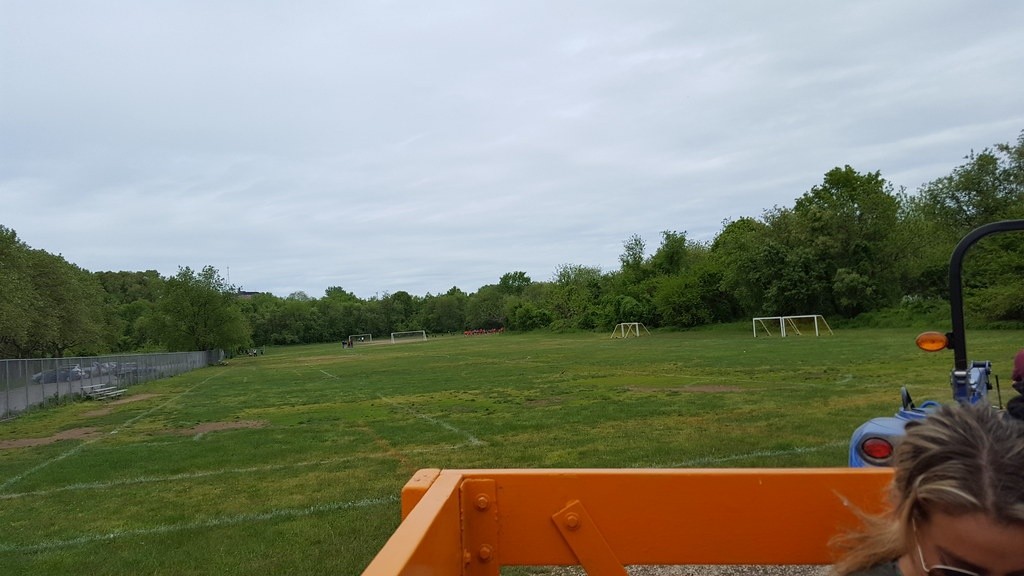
[913,520,978,576]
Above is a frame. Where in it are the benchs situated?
[81,383,128,401]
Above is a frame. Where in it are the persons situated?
[837,398,1024,576]
[342,340,347,349]
[1006,350,1024,419]
[362,337,364,342]
[348,337,354,348]
[464,327,504,337]
[253,350,257,356]
